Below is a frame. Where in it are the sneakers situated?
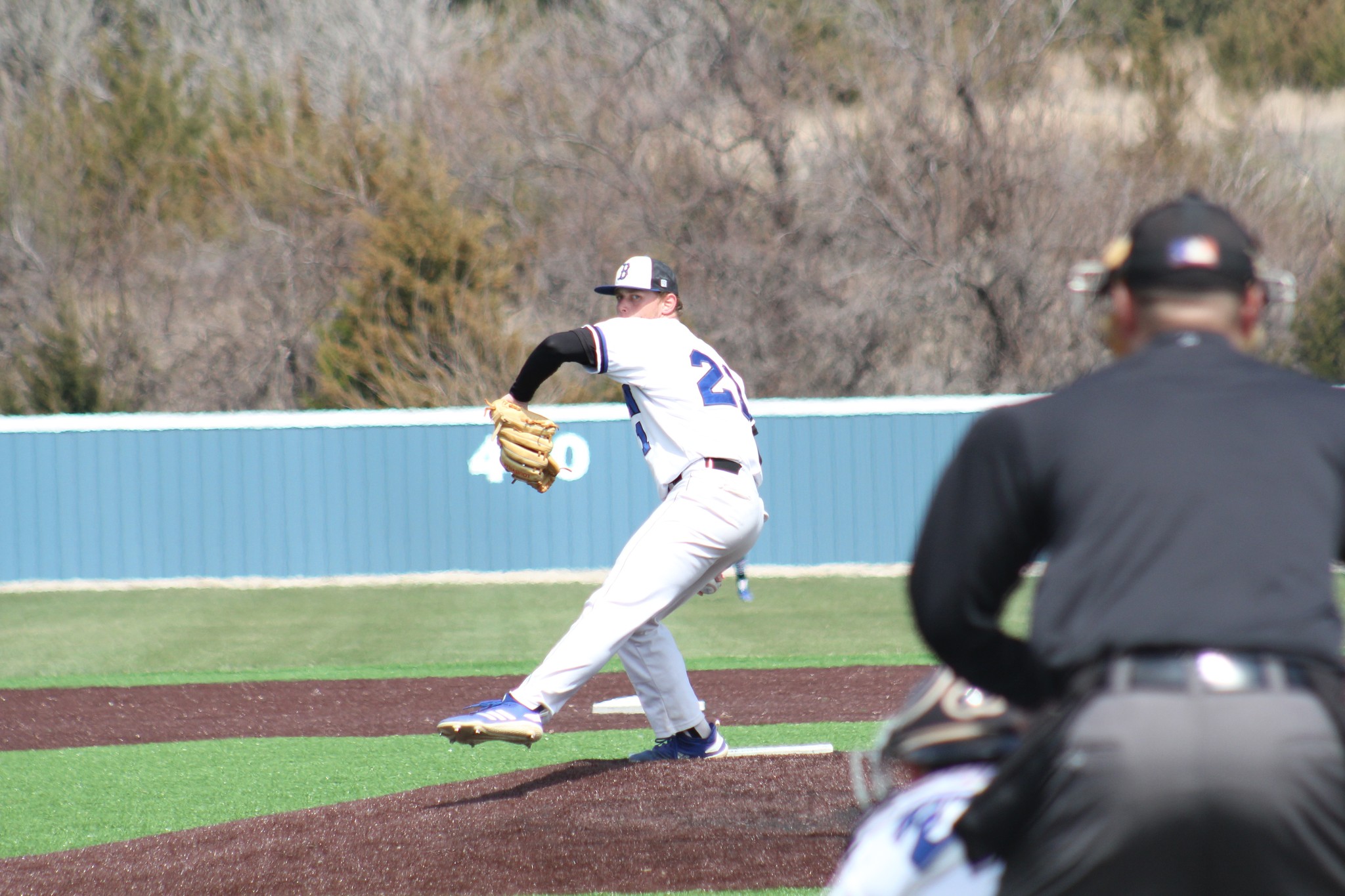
[629,722,727,763]
[437,692,543,749]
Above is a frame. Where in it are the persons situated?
[436,256,768,760]
[905,189,1345,896]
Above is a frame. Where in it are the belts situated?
[668,458,740,493]
[1068,652,1309,692]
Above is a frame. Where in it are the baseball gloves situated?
[482,396,573,494]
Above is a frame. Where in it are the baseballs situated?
[700,579,721,595]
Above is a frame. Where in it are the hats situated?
[1094,192,1258,291]
[594,255,679,295]
